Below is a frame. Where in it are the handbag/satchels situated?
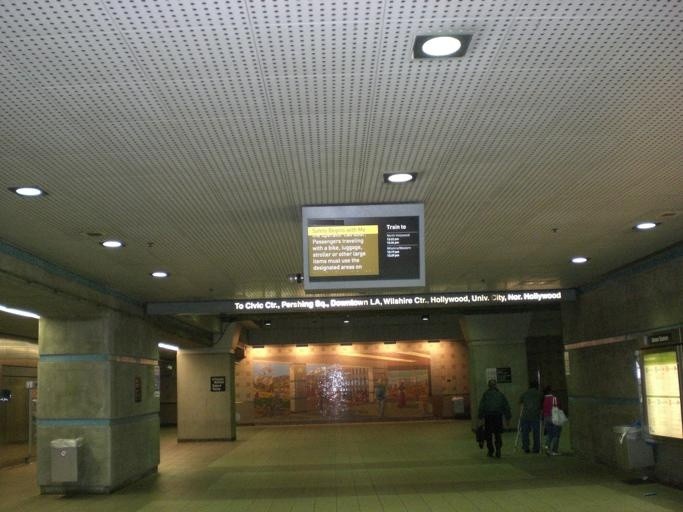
[475,426,486,442]
[550,406,569,427]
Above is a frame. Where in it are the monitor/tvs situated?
[301,202,427,291]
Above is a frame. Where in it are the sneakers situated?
[523,447,562,457]
[487,448,501,458]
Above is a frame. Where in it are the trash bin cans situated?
[613,424,655,470]
[452,397,465,415]
[50,437,87,483]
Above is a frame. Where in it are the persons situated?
[520,378,544,453]
[398,382,406,409]
[477,379,512,459]
[375,378,387,419]
[542,385,562,456]
